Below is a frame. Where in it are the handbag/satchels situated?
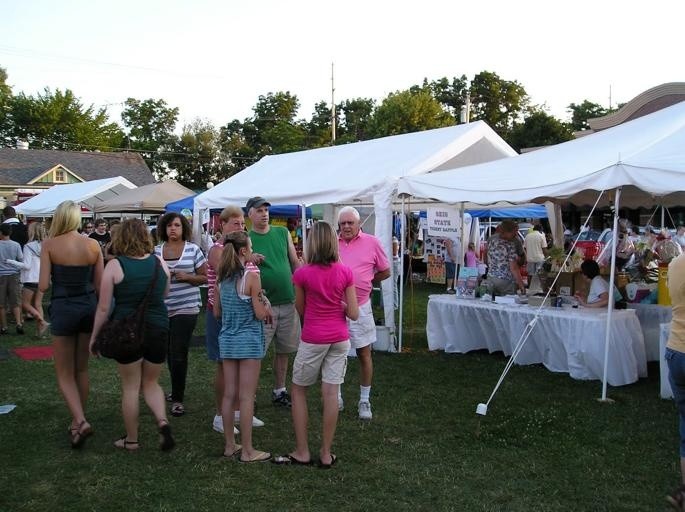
[92,314,144,357]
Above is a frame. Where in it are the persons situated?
[1,207,48,339]
[39,195,401,469]
[443,219,685,310]
[665,251,685,510]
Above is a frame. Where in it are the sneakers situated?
[235,411,264,426]
[2,327,9,333]
[321,396,344,411]
[666,485,685,511]
[16,324,24,334]
[271,391,292,408]
[165,393,172,401]
[212,415,239,434]
[358,402,372,420]
[172,403,185,415]
[448,288,456,293]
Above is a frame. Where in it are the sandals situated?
[114,435,139,451]
[157,419,175,451]
[70,420,92,449]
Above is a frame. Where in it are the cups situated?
[573,300,578,308]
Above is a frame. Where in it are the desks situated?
[426,294,649,386]
[659,320,676,399]
[627,303,672,362]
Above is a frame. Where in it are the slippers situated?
[38,321,51,338]
[318,452,337,469]
[271,454,311,464]
[223,443,242,457]
[239,450,271,462]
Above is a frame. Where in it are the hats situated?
[246,197,271,212]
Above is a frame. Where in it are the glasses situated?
[341,221,354,225]
